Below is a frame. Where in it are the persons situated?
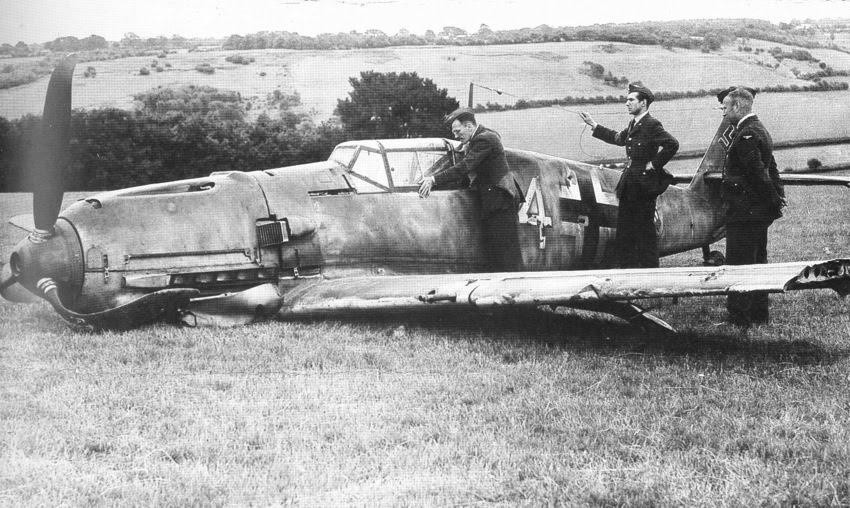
[416,108,527,272]
[720,88,788,326]
[580,83,678,270]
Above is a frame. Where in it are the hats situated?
[717,86,758,102]
[627,82,654,101]
[442,105,475,123]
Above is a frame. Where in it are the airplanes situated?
[0,52,850,335]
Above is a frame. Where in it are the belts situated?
[626,160,647,168]
